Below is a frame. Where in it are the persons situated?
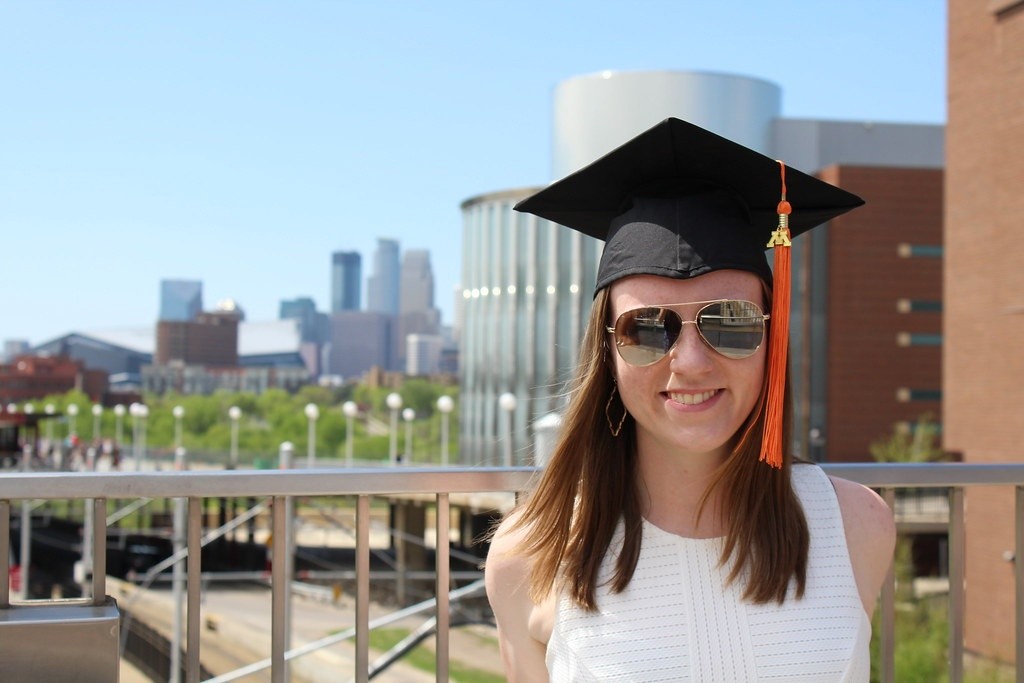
[65,431,123,468]
[659,308,682,354]
[617,311,633,347]
[484,117,897,683]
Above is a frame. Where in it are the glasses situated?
[607,299,771,367]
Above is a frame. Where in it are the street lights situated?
[93,404,103,442]
[436,393,452,466]
[173,404,184,458]
[130,404,148,458]
[498,392,517,466]
[402,407,414,466]
[342,402,357,465]
[114,403,125,448]
[304,403,319,468]
[386,393,401,464]
[229,405,241,465]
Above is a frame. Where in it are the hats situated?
[513,117,867,469]
